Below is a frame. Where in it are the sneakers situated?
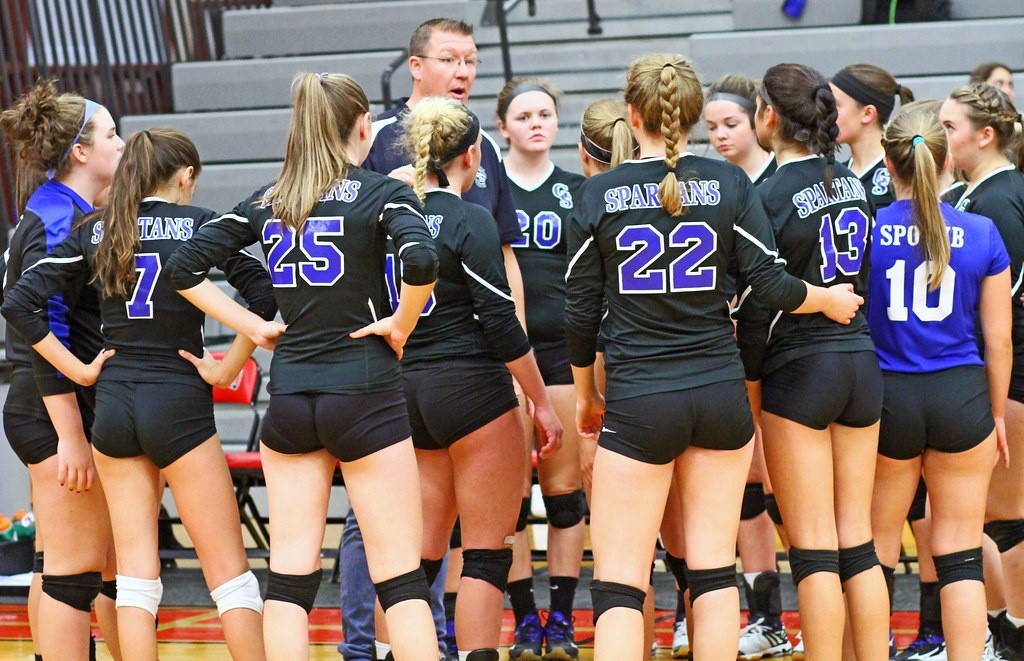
[988,610,1024,661]
[737,614,793,660]
[889,628,898,658]
[541,608,579,661]
[508,612,545,661]
[889,628,948,661]
[671,617,689,658]
[982,625,1001,661]
[791,630,805,660]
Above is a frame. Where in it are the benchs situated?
[120,0,1024,522]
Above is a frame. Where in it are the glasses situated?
[419,53,483,72]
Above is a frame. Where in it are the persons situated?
[0,63,1024,661]
[334,17,529,661]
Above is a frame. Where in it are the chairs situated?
[163,351,351,579]
